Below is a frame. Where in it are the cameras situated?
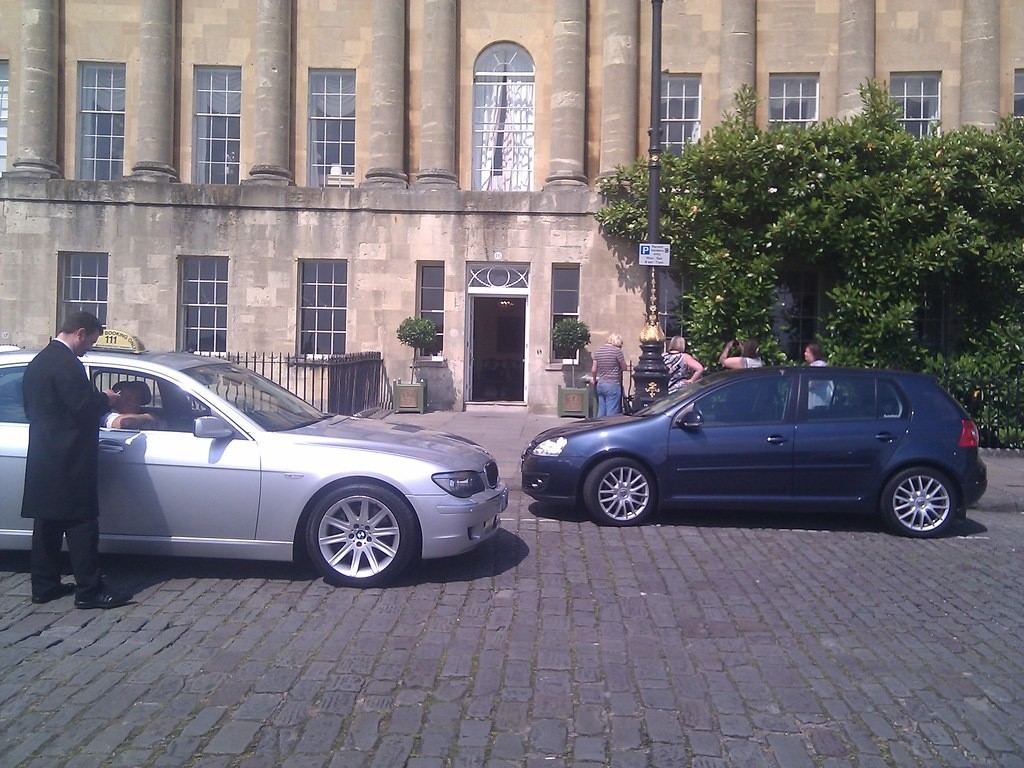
[733,341,739,347]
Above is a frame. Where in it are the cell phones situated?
[115,390,121,394]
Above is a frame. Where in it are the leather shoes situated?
[74,589,134,608]
[32,583,74,603]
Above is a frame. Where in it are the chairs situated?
[138,380,152,406]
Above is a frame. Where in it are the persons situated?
[719,340,762,410]
[804,344,834,411]
[591,333,627,417]
[99,381,168,430]
[22,311,133,609]
[661,336,703,395]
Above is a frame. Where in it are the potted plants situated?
[552,319,590,418]
[393,316,436,414]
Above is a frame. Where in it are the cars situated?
[521,366,988,540]
[0,329,510,588]
